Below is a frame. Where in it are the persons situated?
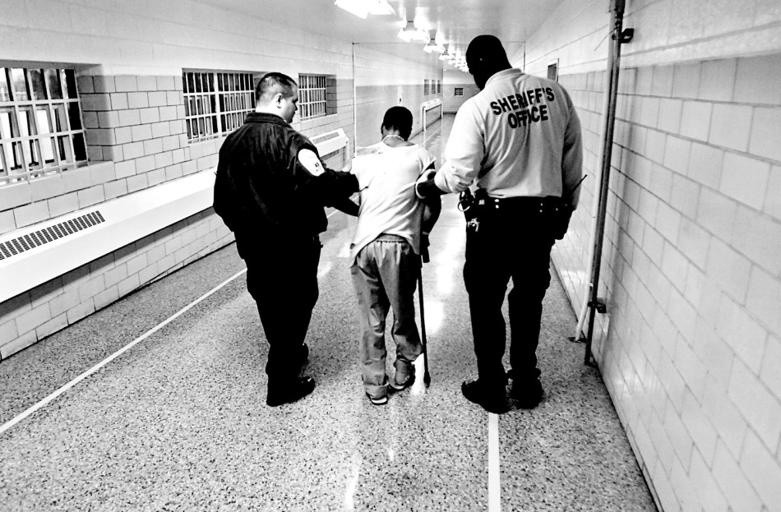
[332,107,440,406]
[413,33,584,414]
[213,70,359,408]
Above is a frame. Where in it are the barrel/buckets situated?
[389,377,405,390]
[371,395,387,405]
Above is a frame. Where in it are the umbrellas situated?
[513,388,542,410]
[302,343,308,364]
[268,376,315,406]
[461,379,509,413]
